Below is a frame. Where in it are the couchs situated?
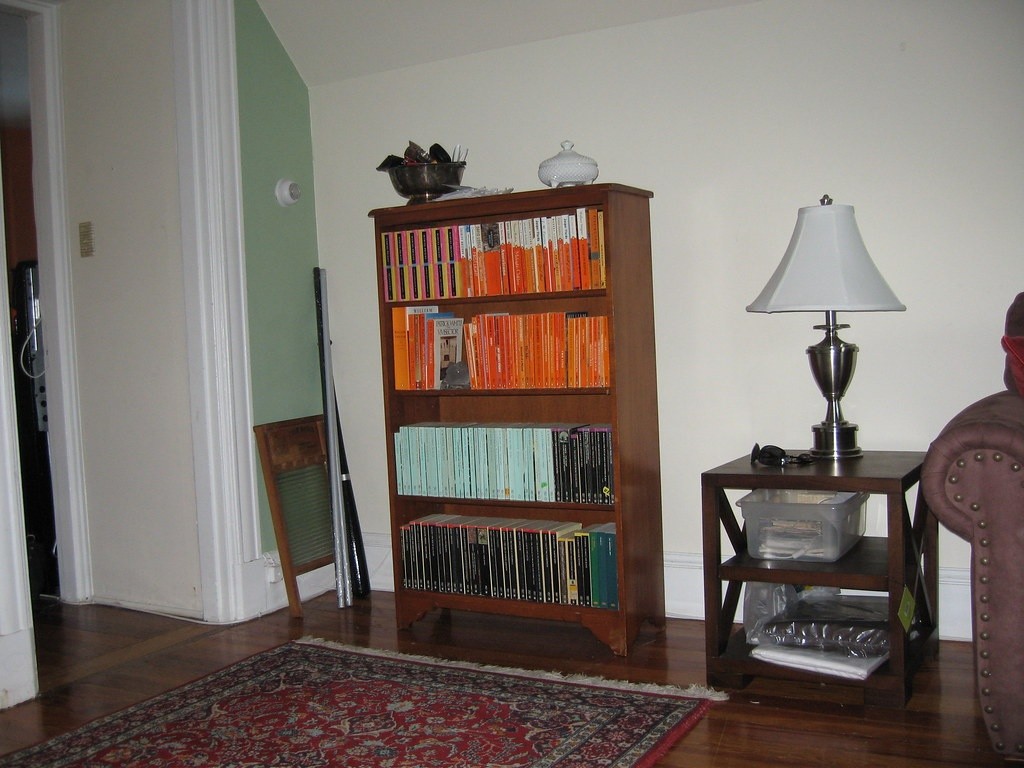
[922,391,1024,768]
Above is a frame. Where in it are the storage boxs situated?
[735,486,871,563]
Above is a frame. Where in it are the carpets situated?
[2,633,727,765]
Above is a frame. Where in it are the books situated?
[394,422,615,504]
[463,311,610,390]
[392,305,465,391]
[381,208,606,302]
[400,514,618,610]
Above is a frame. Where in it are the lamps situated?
[746,194,908,462]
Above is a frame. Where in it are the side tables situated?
[696,450,932,708]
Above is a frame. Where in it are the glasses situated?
[751,443,817,468]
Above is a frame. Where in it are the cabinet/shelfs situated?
[369,184,664,662]
